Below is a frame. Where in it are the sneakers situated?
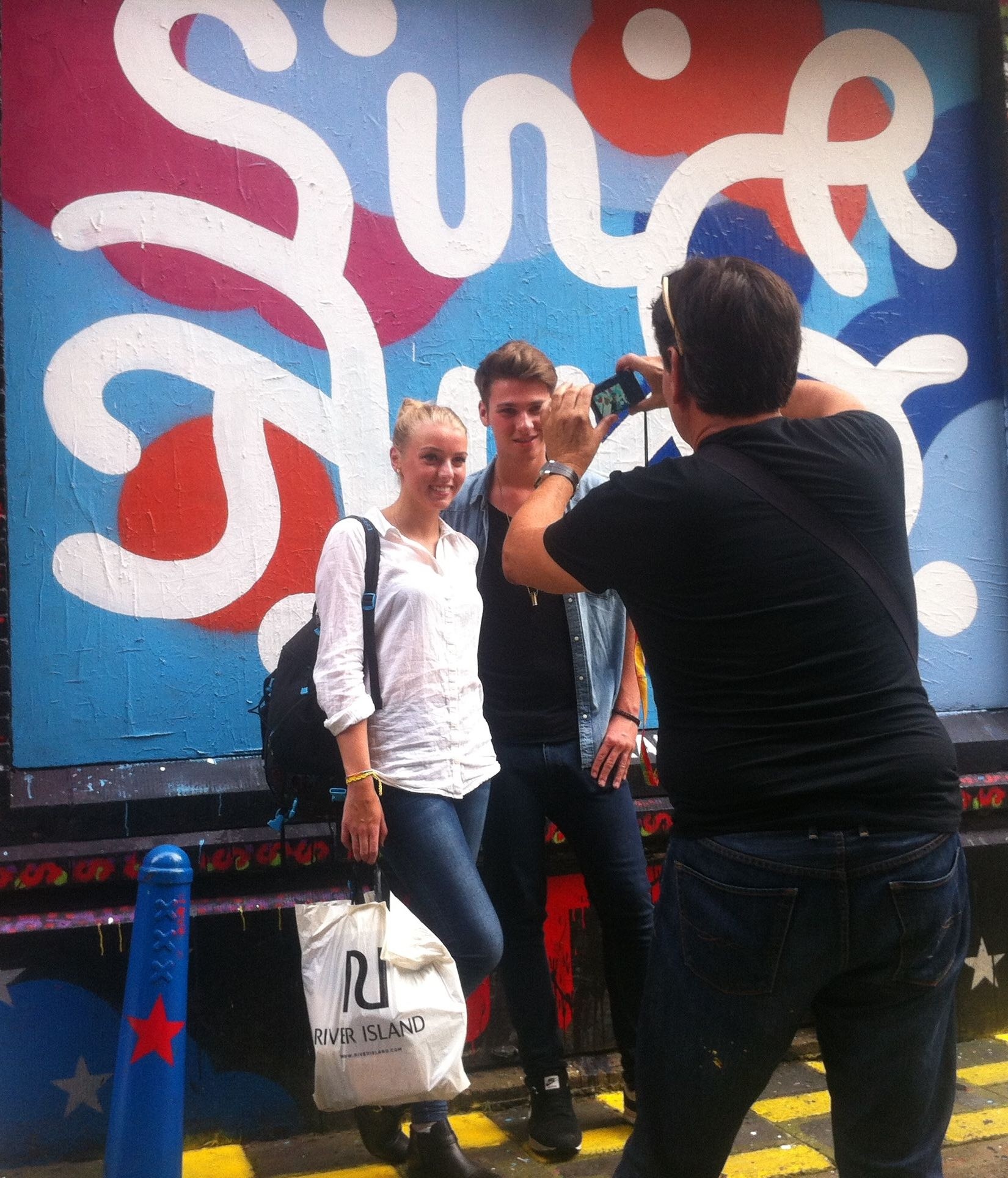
[522,1061,581,1155]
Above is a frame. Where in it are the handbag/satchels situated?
[293,854,468,1114]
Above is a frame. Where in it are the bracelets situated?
[345,769,382,796]
[612,708,640,727]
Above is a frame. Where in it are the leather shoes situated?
[355,1096,413,1161]
[405,1118,498,1178]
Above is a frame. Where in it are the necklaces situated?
[491,464,539,607]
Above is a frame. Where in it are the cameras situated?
[591,370,644,420]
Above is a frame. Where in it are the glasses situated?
[659,267,685,357]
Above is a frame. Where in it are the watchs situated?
[533,458,580,498]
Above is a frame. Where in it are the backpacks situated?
[260,516,383,821]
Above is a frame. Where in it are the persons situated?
[440,340,661,1157]
[311,393,504,1178]
[596,391,622,419]
[502,254,969,1176]
